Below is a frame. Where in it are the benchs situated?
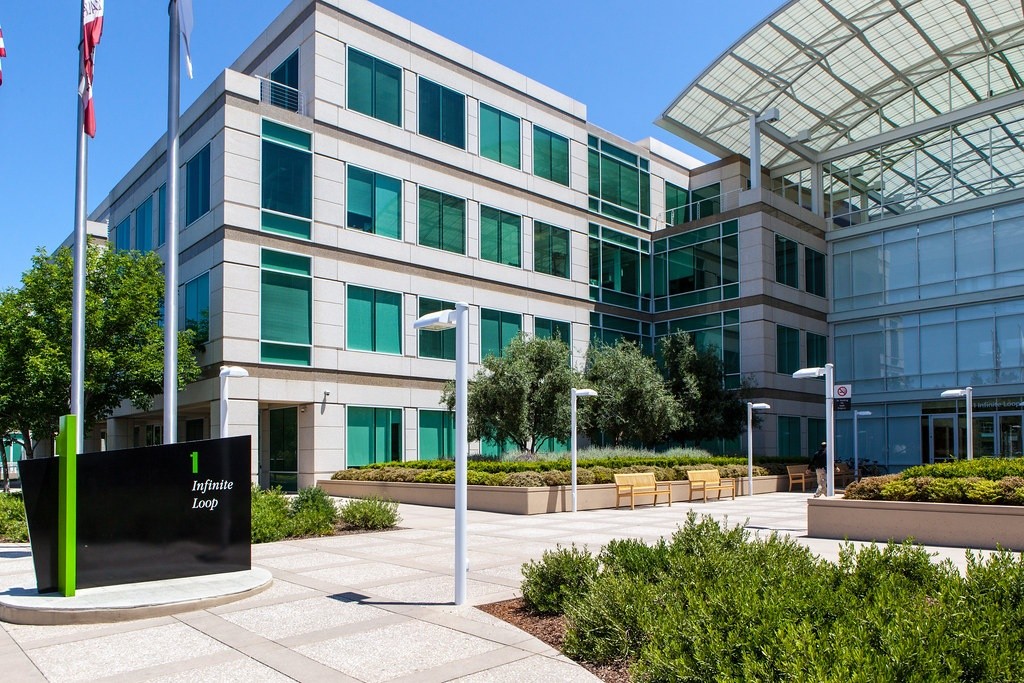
[613,472,672,510]
[787,465,817,493]
[687,469,736,503]
[834,462,862,489]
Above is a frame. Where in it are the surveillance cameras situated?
[324,390,330,395]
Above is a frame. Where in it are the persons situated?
[805,442,840,498]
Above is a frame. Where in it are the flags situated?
[76,0,106,138]
[0,25,6,85]
[176,0,195,78]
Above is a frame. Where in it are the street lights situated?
[854,410,871,481]
[413,302,469,606]
[792,363,834,497]
[1010,425,1021,457]
[219,365,249,438]
[747,402,770,497]
[571,388,598,512]
[941,387,973,461]
